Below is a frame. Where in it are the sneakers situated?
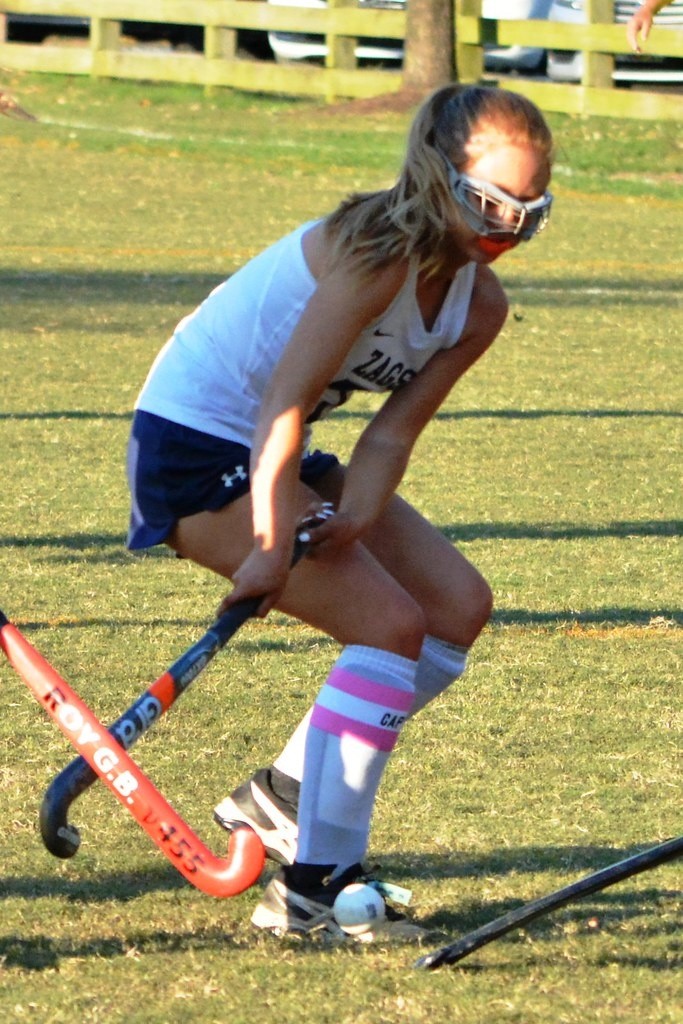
[250,863,429,945]
[213,768,300,867]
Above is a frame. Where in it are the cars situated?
[268,0,555,70]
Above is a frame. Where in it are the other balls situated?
[333,881,387,937]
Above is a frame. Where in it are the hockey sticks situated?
[1,609,269,900]
[411,835,683,974]
[37,506,314,860]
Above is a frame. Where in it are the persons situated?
[627,0,672,53]
[126,82,553,942]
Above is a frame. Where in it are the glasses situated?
[450,171,552,242]
